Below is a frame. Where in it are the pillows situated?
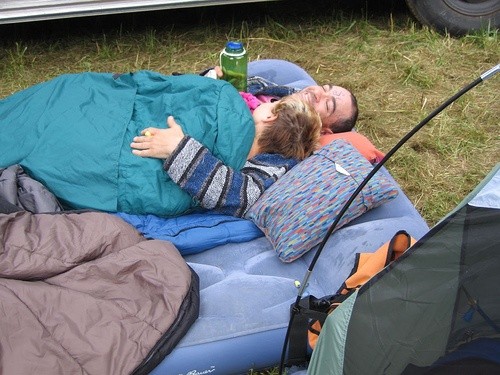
[244,135,399,264]
[311,128,390,167]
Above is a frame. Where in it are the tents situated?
[277,64,500,375]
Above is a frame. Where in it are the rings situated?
[145,126,153,136]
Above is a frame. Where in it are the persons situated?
[246,99,322,164]
[128,68,360,219]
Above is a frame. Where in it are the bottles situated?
[204,40,248,94]
